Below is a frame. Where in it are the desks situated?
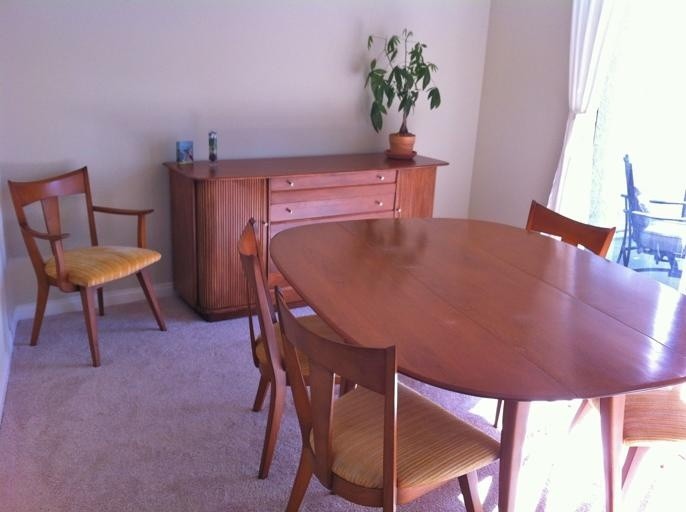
[269,217,686,512]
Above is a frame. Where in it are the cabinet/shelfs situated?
[162,154,449,323]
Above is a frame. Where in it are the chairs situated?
[7,166,166,367]
[616,155,686,272]
[570,383,686,494]
[526,199,616,257]
[237,219,356,480]
[274,286,501,512]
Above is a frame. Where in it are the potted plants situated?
[365,28,441,159]
